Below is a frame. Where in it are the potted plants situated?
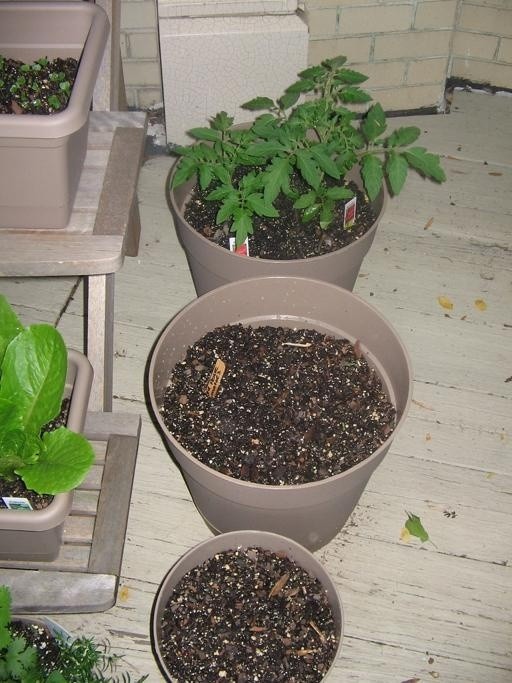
[165,57,446,303]
[3,298,93,564]
[0,589,146,683]
[2,3,111,227]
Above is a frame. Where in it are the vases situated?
[148,276,411,683]
